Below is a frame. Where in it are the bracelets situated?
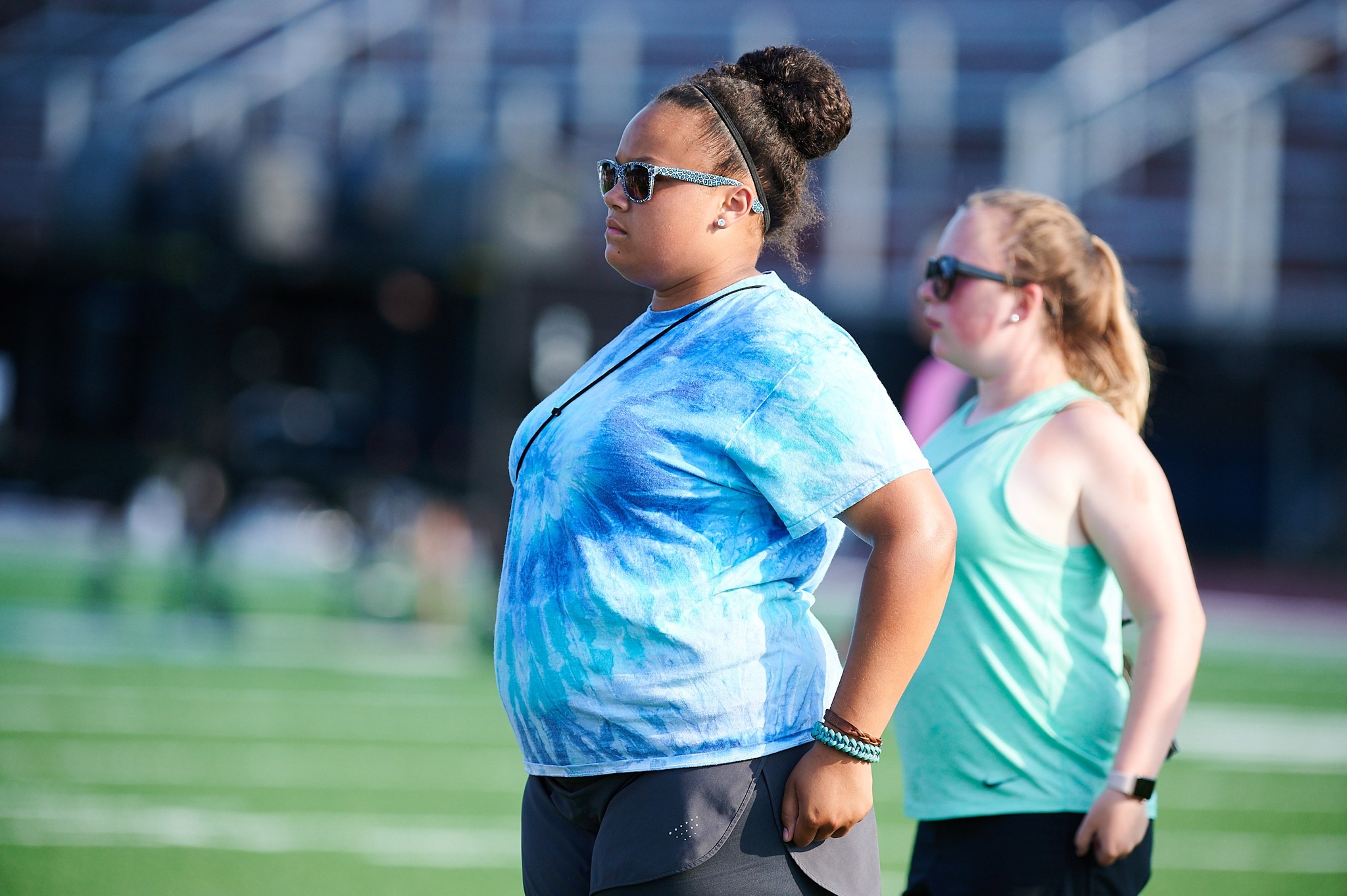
[809,720,881,763]
[824,707,883,748]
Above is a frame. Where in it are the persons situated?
[895,190,1206,896]
[492,38,961,896]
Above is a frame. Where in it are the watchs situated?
[1107,773,1157,801]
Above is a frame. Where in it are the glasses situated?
[596,158,765,214]
[926,255,1057,318]
